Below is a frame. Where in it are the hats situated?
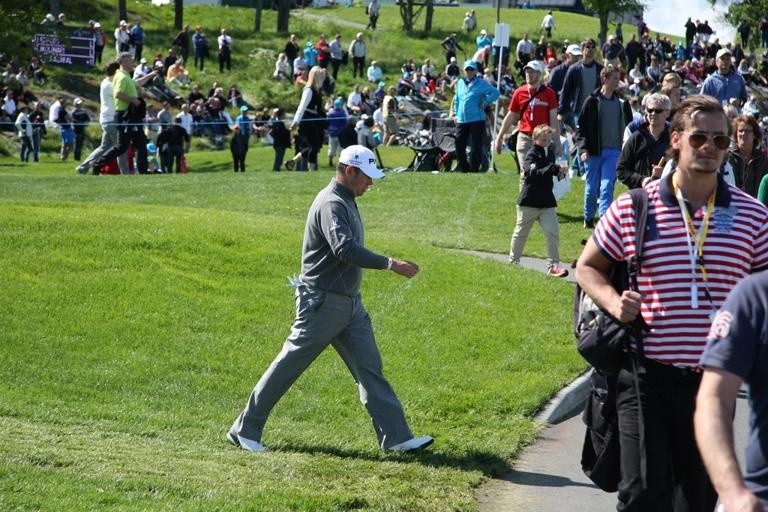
[119,20,128,26]
[45,13,55,22]
[155,61,164,68]
[523,60,542,73]
[73,97,82,107]
[338,145,386,180]
[565,43,583,55]
[464,60,478,70]
[94,23,101,29]
[140,58,147,64]
[716,48,732,61]
[334,99,343,111]
[239,106,249,111]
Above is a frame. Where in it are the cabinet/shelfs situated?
[34,36,96,67]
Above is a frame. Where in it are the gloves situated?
[286,273,309,289]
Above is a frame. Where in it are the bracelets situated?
[386,257,392,271]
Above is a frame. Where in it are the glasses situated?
[465,68,475,71]
[678,131,732,150]
[646,106,669,114]
[586,46,595,48]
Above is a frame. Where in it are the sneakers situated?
[546,262,568,277]
[283,160,295,171]
[226,430,273,453]
[584,218,595,229]
[381,436,434,458]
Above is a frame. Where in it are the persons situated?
[689,274,768,511]
[506,125,570,278]
[366,0,381,31]
[578,95,768,511]
[1,10,768,230]
[228,144,433,453]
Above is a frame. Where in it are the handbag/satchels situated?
[574,260,635,366]
[504,129,519,152]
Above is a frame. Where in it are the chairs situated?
[404,125,456,174]
